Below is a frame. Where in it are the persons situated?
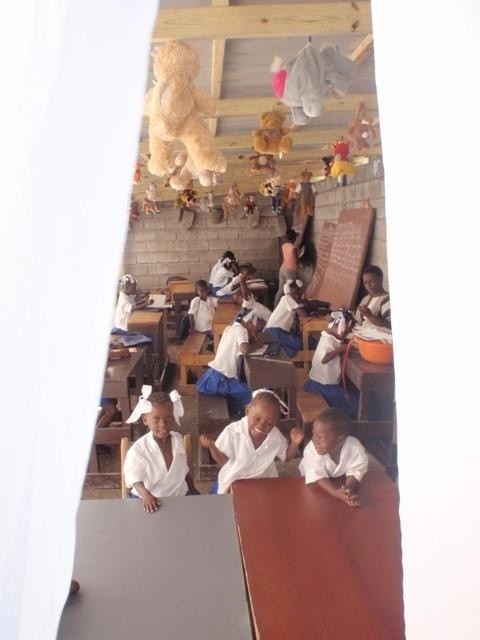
[110,274,154,335]
[299,408,368,506]
[200,393,305,494]
[97,397,118,428]
[123,392,199,513]
[304,265,391,414]
[187,229,305,414]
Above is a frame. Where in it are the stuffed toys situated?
[129,41,376,221]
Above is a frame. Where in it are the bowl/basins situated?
[351,335,393,364]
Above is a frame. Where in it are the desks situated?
[56,493,254,640]
[82,276,398,500]
[230,469,405,640]
